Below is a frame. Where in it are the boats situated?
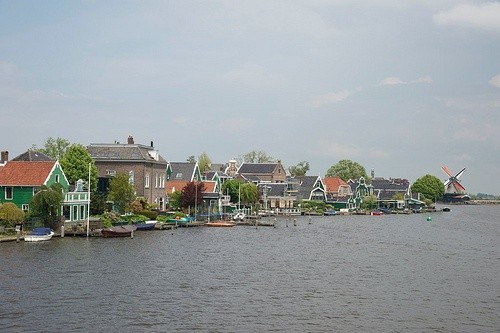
[23,226,55,241]
[306,207,450,215]
[135,223,155,230]
[206,220,238,226]
[100,224,138,237]
